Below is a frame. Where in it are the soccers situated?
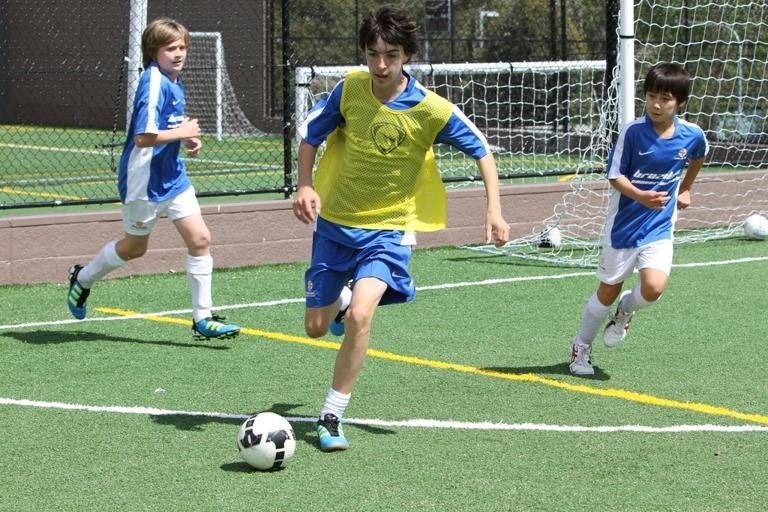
[743,214,768,240]
[541,225,562,249]
[237,411,297,471]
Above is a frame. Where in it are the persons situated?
[565,63,710,376]
[65,16,241,343]
[289,6,510,453]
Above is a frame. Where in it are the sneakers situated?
[191,316,241,341]
[67,264,90,321]
[570,336,595,375]
[603,290,635,348]
[330,279,353,337]
[316,412,349,450]
